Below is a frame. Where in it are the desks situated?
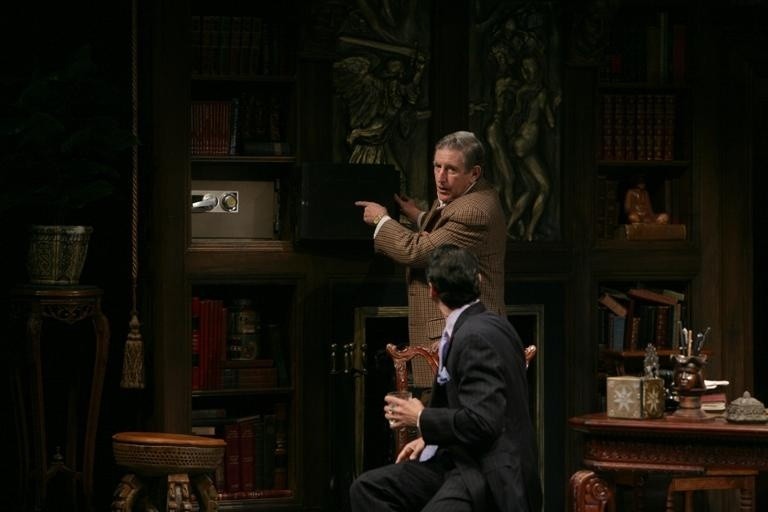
[570,407,768,512]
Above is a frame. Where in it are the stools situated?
[110,431,229,512]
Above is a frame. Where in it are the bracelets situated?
[371,212,387,223]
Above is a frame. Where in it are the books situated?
[669,376,731,413]
[189,294,293,499]
[594,87,688,244]
[187,93,292,156]
[595,282,689,411]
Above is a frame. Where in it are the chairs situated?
[385,342,536,512]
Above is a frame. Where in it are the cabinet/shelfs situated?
[152,0,307,510]
[599,0,702,361]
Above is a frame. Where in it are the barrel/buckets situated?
[25,224,93,288]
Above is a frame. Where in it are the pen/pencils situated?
[677,321,712,356]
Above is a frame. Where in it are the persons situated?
[348,247,536,511]
[485,50,526,235]
[353,128,508,409]
[348,52,427,224]
[504,58,562,241]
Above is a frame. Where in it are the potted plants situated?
[0,54,141,285]
